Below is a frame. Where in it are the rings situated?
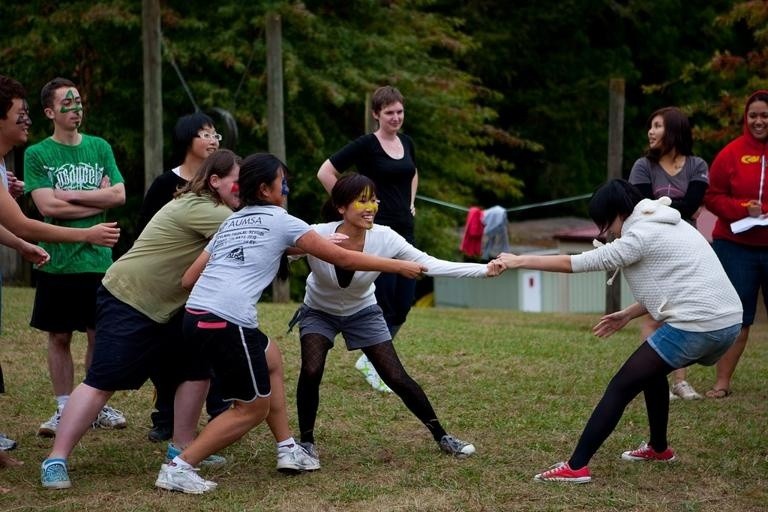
[329,233,335,237]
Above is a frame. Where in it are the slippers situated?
[706,387,733,398]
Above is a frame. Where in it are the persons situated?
[314,84,421,397]
[702,86,768,400]
[491,177,743,486]
[37,147,352,490]
[6,168,27,202]
[624,107,711,405]
[0,436,19,451]
[150,150,429,497]
[136,111,235,447]
[286,169,508,463]
[0,74,122,496]
[22,77,130,439]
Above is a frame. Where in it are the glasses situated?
[197,132,223,141]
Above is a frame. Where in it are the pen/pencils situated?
[668,183,672,196]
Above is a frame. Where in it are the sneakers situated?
[355,356,395,392]
[534,462,592,483]
[92,404,128,430]
[621,445,675,463]
[671,380,702,399]
[146,422,227,495]
[37,407,61,437]
[275,442,321,473]
[41,457,72,489]
[0,434,18,450]
[439,436,476,459]
